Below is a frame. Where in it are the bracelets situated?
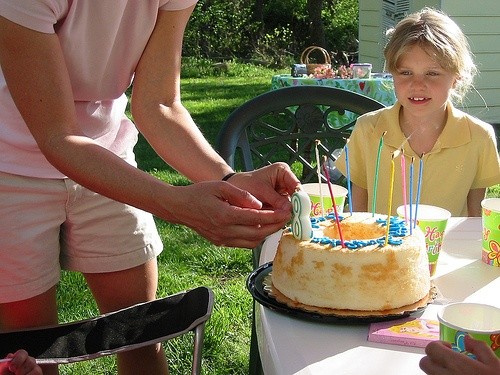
[222,173,237,182]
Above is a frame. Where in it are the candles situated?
[290,191,312,241]
[315,131,425,248]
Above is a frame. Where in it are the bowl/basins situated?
[352,63,372,79]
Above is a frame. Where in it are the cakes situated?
[271,189,431,311]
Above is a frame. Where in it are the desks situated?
[249,217,500,375]
[271,74,398,185]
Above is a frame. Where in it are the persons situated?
[335,7,500,217]
[0,350,43,375]
[0,0,306,375]
[419,334,500,375]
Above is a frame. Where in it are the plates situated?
[245,262,438,323]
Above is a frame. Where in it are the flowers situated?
[308,64,362,79]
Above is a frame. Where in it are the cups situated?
[396,204,451,277]
[481,198,500,267]
[302,182,349,217]
[437,301,500,363]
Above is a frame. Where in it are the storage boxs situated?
[353,63,372,79]
[367,317,440,348]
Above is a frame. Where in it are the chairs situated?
[216,86,387,185]
